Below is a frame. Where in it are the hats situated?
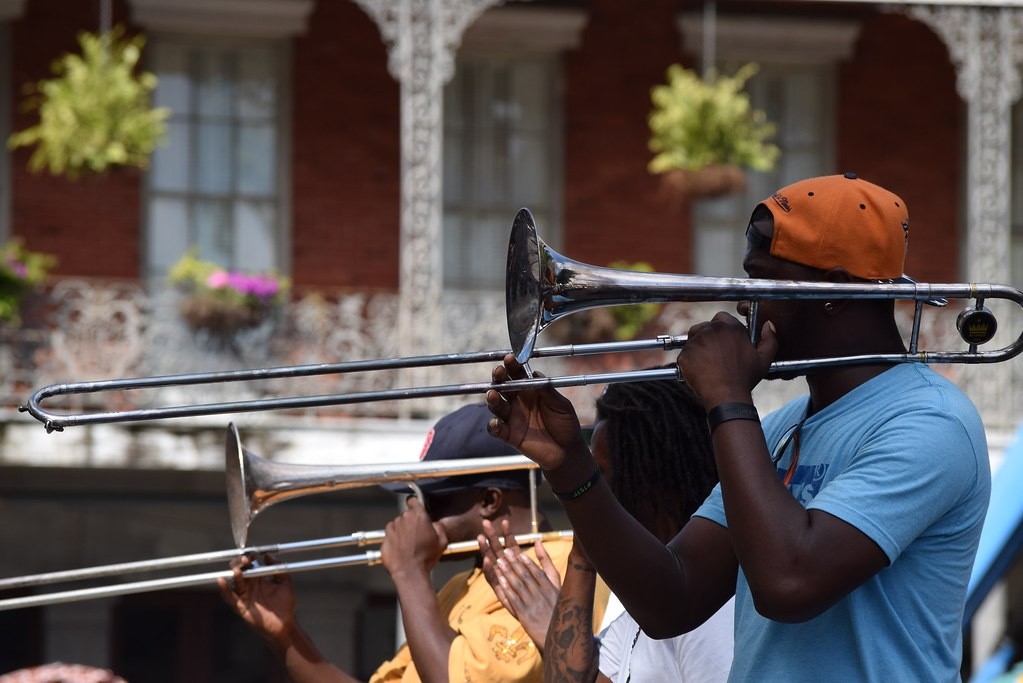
[745,172,948,308]
[378,403,542,491]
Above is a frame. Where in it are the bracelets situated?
[552,464,602,500]
[707,402,761,436]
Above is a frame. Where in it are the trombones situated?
[0,419,573,618]
[17,200,1023,436]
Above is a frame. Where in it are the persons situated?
[487,174,992,683]
[477,361,736,683]
[217,403,609,683]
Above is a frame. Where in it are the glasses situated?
[426,486,487,523]
[772,417,806,490]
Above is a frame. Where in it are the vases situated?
[182,299,264,334]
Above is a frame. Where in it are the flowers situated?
[171,252,293,308]
[0,234,61,325]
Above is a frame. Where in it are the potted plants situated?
[8,26,174,188]
[647,48,778,197]
